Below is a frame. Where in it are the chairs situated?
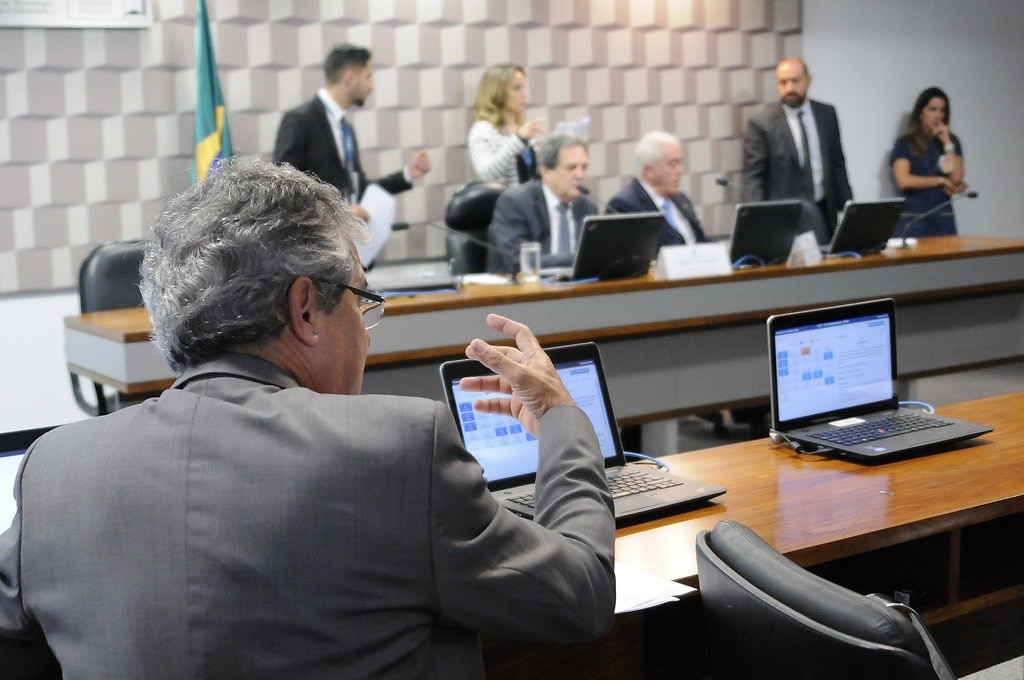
[68,195,957,680]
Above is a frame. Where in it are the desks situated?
[62,236,1024,429]
[590,389,1024,680]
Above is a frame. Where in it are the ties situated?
[662,198,677,231]
[340,119,354,172]
[558,203,570,256]
[798,112,814,195]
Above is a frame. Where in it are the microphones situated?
[902,191,978,248]
[392,219,520,283]
[576,185,617,215]
[715,178,760,201]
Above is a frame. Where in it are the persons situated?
[604,131,709,258]
[274,47,431,270]
[739,58,853,236]
[0,159,617,680]
[468,63,546,188]
[888,87,968,237]
[485,131,599,273]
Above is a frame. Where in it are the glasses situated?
[285,276,386,330]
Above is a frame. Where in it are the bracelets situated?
[945,143,954,151]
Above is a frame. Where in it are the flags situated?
[192,0,233,186]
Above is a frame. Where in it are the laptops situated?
[826,199,906,255]
[766,298,994,464]
[540,212,667,283]
[729,201,803,268]
[440,342,726,521]
